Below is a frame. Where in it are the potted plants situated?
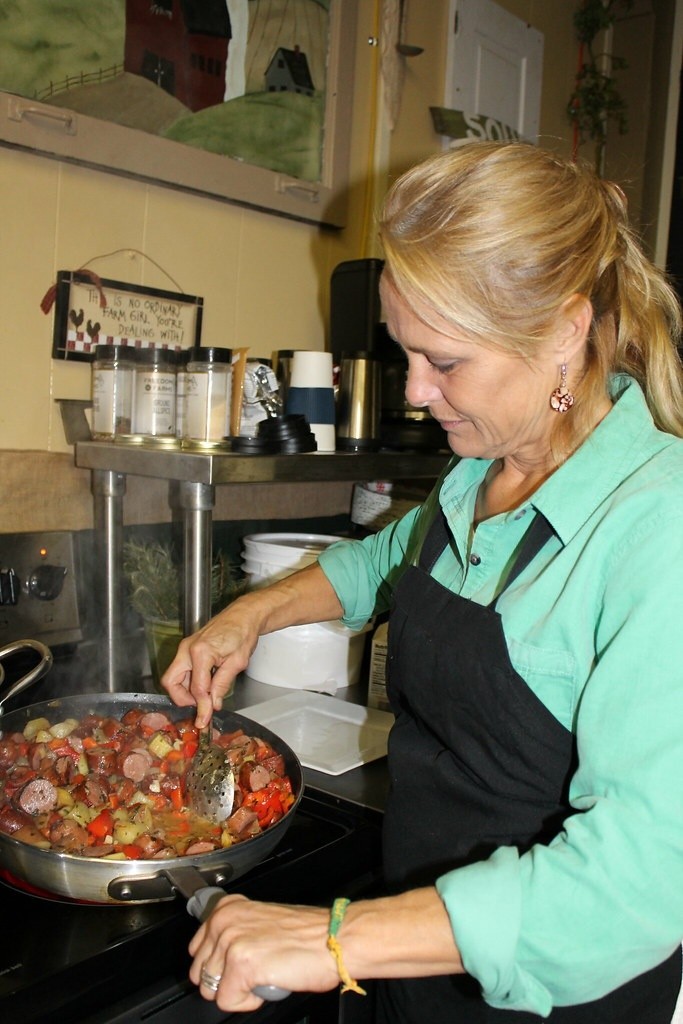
[122,535,252,699]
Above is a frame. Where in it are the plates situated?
[233,691,395,776]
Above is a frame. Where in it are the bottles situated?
[180,347,233,453]
[132,348,175,437]
[90,345,136,441]
[174,350,190,439]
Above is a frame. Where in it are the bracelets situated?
[327,897,366,996]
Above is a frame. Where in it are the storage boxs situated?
[368,621,390,704]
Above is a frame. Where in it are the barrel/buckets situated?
[242,532,374,692]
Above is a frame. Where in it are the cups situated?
[335,350,382,454]
[286,351,337,455]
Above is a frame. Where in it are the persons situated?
[161,140,683,1024]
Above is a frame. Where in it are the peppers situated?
[7,726,296,859]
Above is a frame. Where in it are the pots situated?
[0,692,305,1001]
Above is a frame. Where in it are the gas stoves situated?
[0,785,392,1024]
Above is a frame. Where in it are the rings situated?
[200,968,222,992]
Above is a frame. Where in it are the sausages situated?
[0,708,270,860]
[259,753,286,778]
